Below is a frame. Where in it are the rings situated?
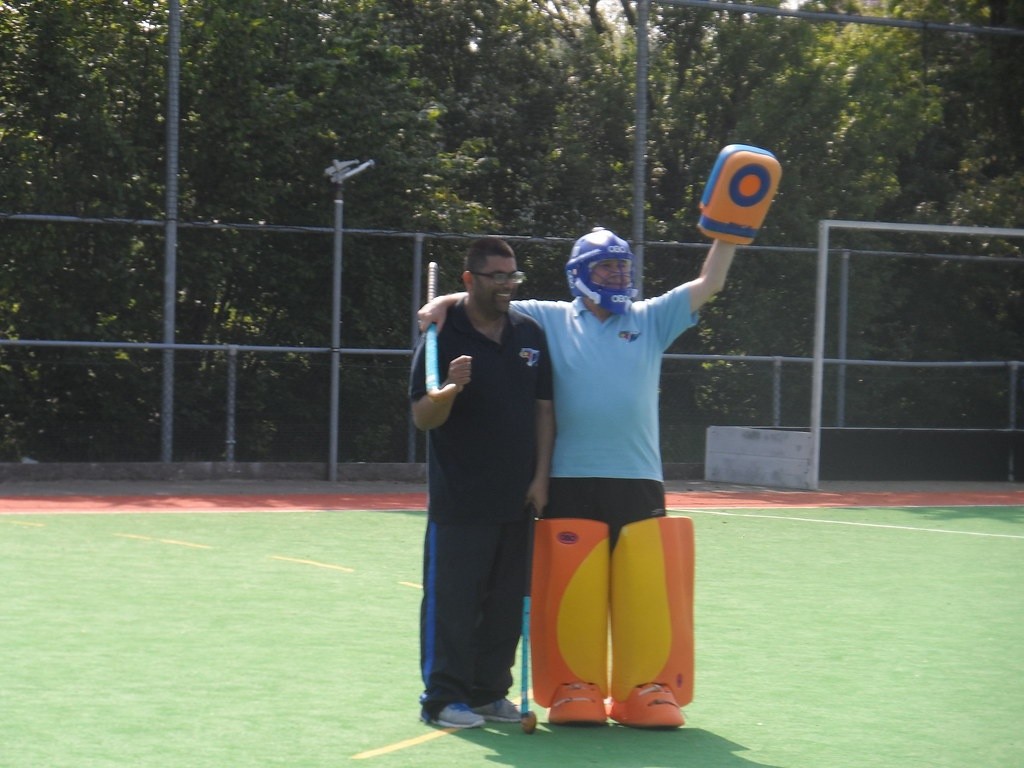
[417,319,422,322]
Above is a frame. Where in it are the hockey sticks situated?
[423,259,457,402]
[520,501,538,735]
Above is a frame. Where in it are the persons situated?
[414,144,781,728]
[407,237,557,729]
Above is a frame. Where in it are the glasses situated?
[472,270,526,284]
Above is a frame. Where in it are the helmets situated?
[565,226,639,314]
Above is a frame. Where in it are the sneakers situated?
[472,698,521,722]
[420,702,486,728]
